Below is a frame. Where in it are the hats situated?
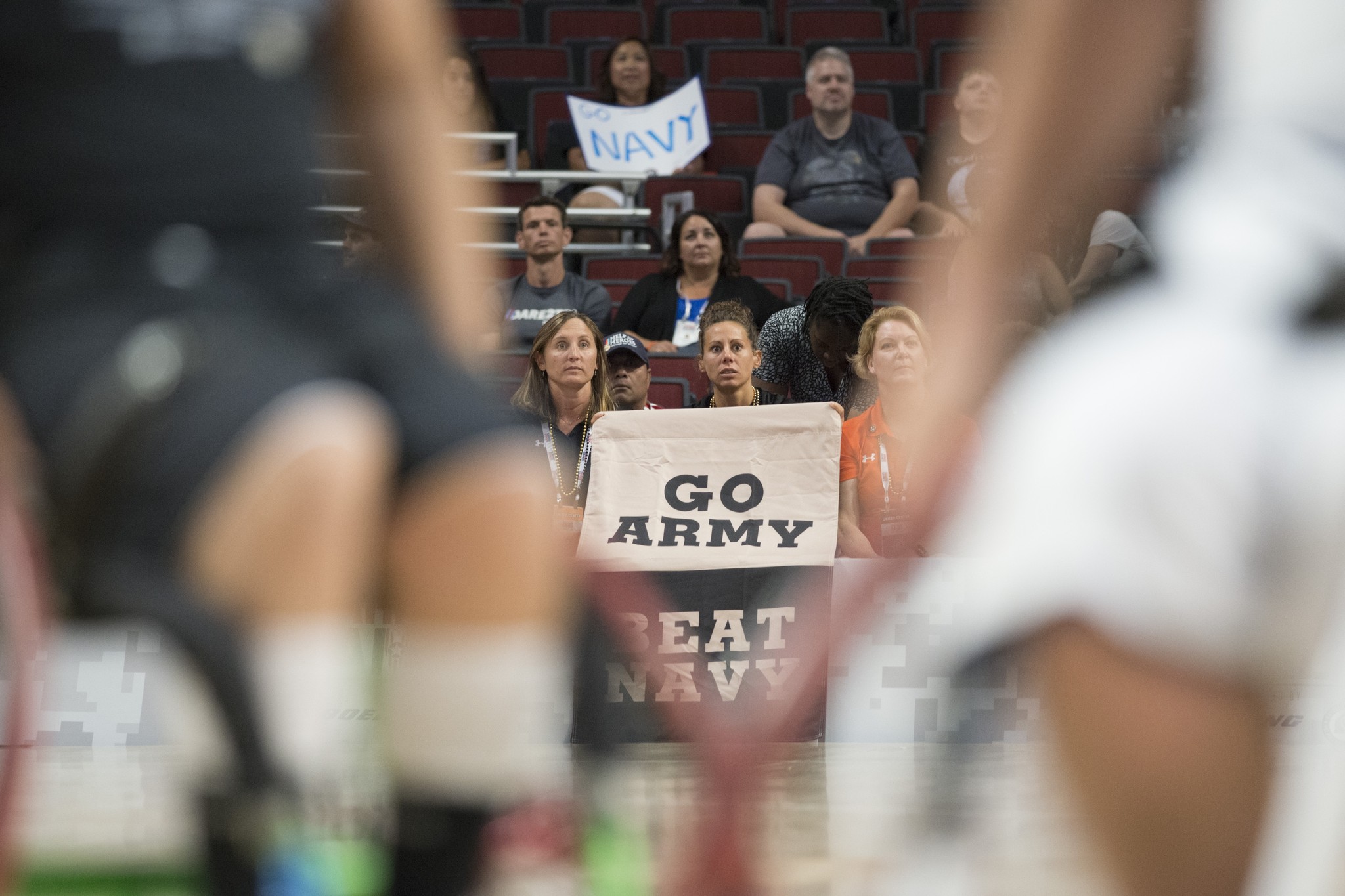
[603,332,649,364]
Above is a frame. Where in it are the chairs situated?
[440,0,1011,440]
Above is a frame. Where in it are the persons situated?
[0,1,572,895]
[717,0,1343,895]
[349,33,1156,742]
